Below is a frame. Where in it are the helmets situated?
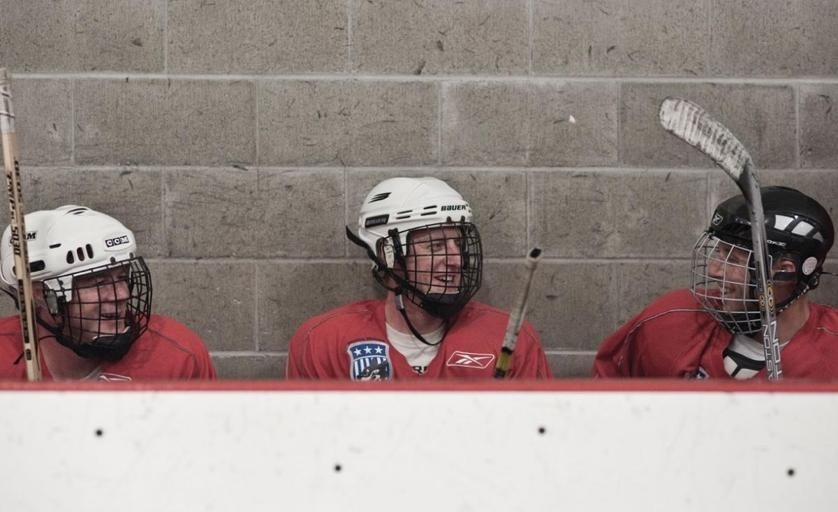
[358,176,483,319]
[687,185,834,336]
[1,205,152,361]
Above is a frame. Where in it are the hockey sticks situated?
[660,93,781,382]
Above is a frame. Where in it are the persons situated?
[586,185,837,380]
[284,177,555,380]
[0,205,218,383]
